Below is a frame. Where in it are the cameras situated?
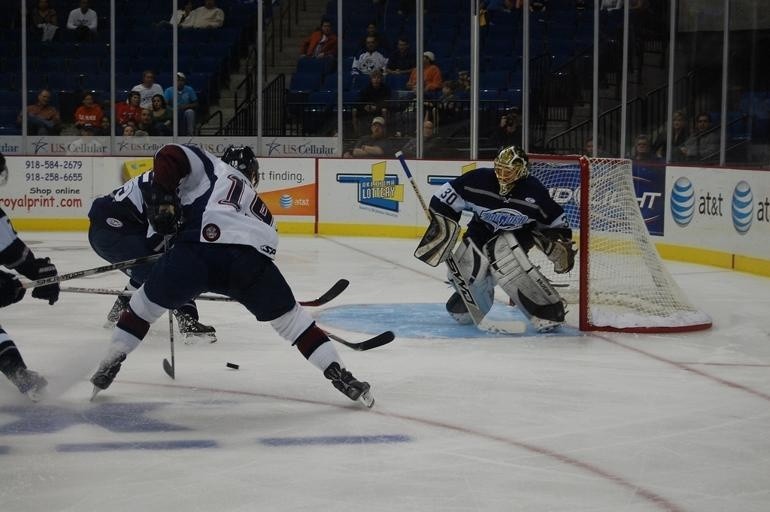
[506,116,513,127]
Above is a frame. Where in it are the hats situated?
[423,51,435,62]
[371,116,386,126]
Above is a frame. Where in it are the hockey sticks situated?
[56,278,349,306]
[324,330,394,351]
[163,307,176,379]
[395,151,527,334]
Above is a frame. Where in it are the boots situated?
[171,297,215,334]
[322,362,372,401]
[9,365,49,396]
[88,347,130,390]
[106,292,133,323]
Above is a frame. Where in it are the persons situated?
[169,0,225,30]
[90,141,375,408]
[427,144,579,331]
[16,68,202,136]
[584,107,729,165]
[575,0,651,13]
[0,152,60,402]
[307,2,541,159]
[88,168,218,344]
[30,0,98,49]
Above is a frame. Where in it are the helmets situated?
[176,70,187,80]
[493,144,529,182]
[220,142,262,182]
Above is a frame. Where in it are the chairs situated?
[285,0,521,114]
[0,0,271,136]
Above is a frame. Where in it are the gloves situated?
[29,253,65,307]
[142,218,176,253]
[0,272,27,308]
